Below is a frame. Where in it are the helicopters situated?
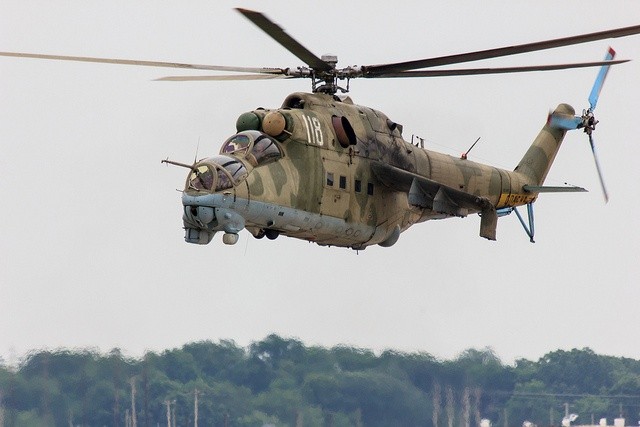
[1,3,639,251]
